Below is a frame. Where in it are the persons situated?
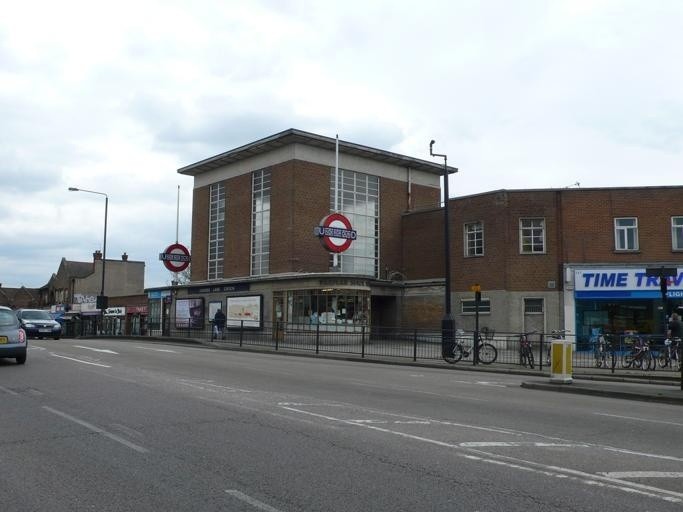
[667,312,682,359]
[213,309,225,340]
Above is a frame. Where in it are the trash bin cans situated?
[550,340,573,384]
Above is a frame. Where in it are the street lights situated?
[68,187,108,335]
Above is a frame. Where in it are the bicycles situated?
[621,336,656,371]
[514,329,538,368]
[442,327,497,364]
[657,337,683,371]
[593,335,617,368]
[547,330,571,367]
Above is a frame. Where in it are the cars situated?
[15,308,61,340]
[0,306,27,363]
